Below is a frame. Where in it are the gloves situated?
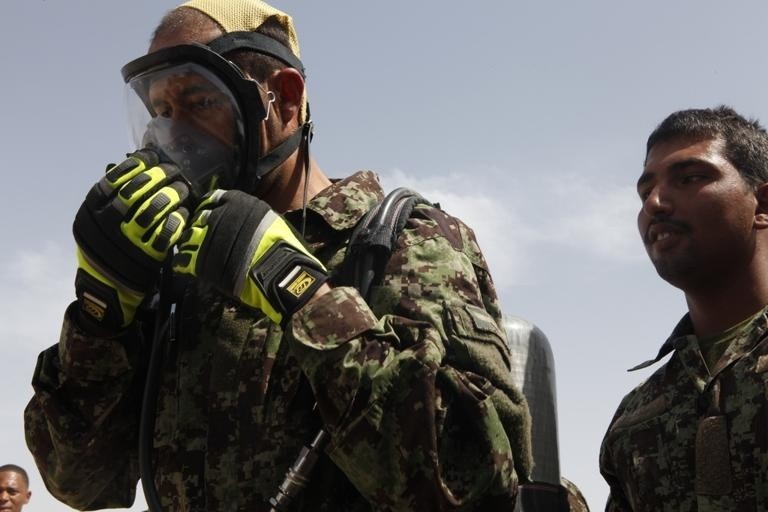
[169,188,333,331]
[76,146,189,329]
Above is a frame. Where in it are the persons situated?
[0,464,33,511]
[23,2,535,510]
[598,104,768,511]
